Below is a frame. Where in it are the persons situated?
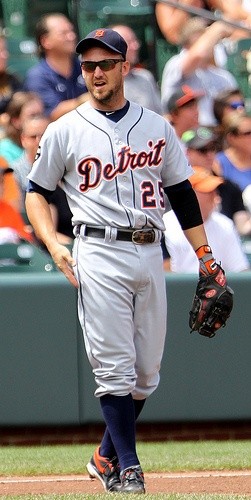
[24,29,233,495]
[0,0,251,274]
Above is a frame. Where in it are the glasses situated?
[224,103,246,111]
[80,59,123,72]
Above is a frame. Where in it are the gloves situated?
[195,245,219,278]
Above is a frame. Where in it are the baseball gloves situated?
[189,245,234,339]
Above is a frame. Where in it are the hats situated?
[167,84,205,113]
[187,165,225,194]
[75,28,127,62]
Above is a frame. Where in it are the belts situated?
[78,224,155,245]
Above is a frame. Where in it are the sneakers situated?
[86,445,121,493]
[120,465,145,495]
